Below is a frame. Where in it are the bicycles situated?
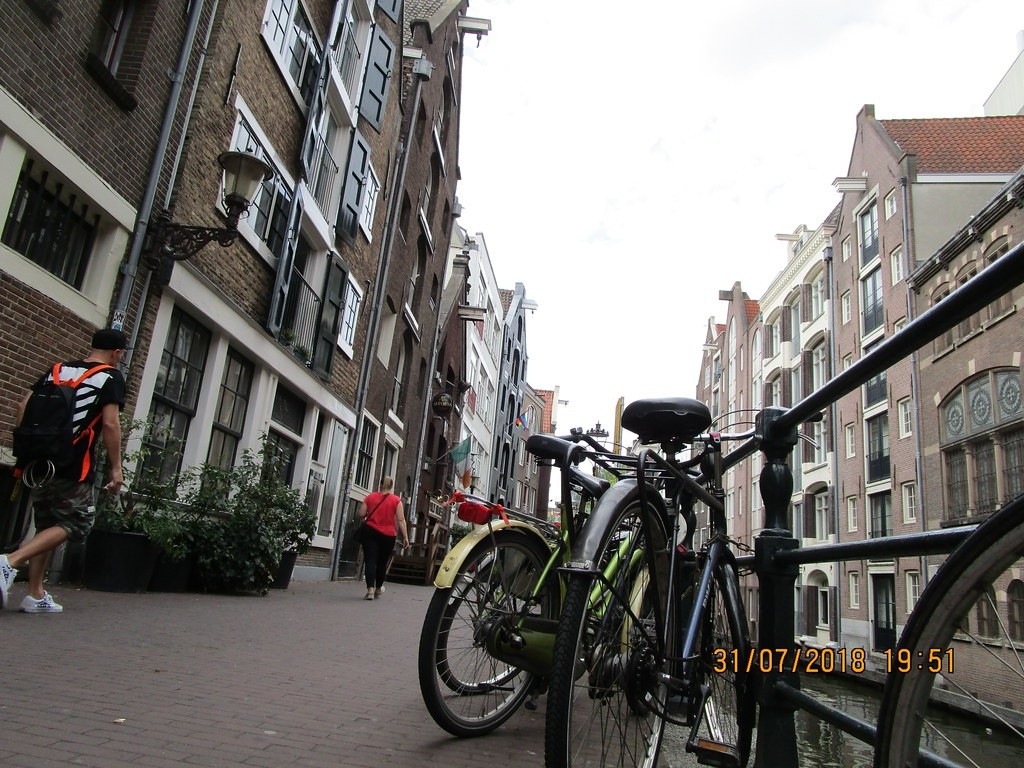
[417,395,826,767]
[874,491,1024,768]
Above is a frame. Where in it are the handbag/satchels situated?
[354,523,366,542]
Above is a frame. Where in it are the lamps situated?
[147,147,273,271]
[457,379,467,394]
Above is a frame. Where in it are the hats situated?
[92,328,134,350]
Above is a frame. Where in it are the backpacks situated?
[9,362,116,471]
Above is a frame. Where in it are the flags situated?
[449,435,473,488]
[513,410,530,431]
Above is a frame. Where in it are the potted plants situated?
[285,328,297,353]
[294,345,310,364]
[61,410,317,597]
[421,457,433,471]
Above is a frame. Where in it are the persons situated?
[355,476,409,599]
[0,329,134,613]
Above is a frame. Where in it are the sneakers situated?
[22,589,64,613]
[0,554,19,608]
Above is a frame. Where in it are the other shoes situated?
[364,593,374,600]
[375,584,386,595]
